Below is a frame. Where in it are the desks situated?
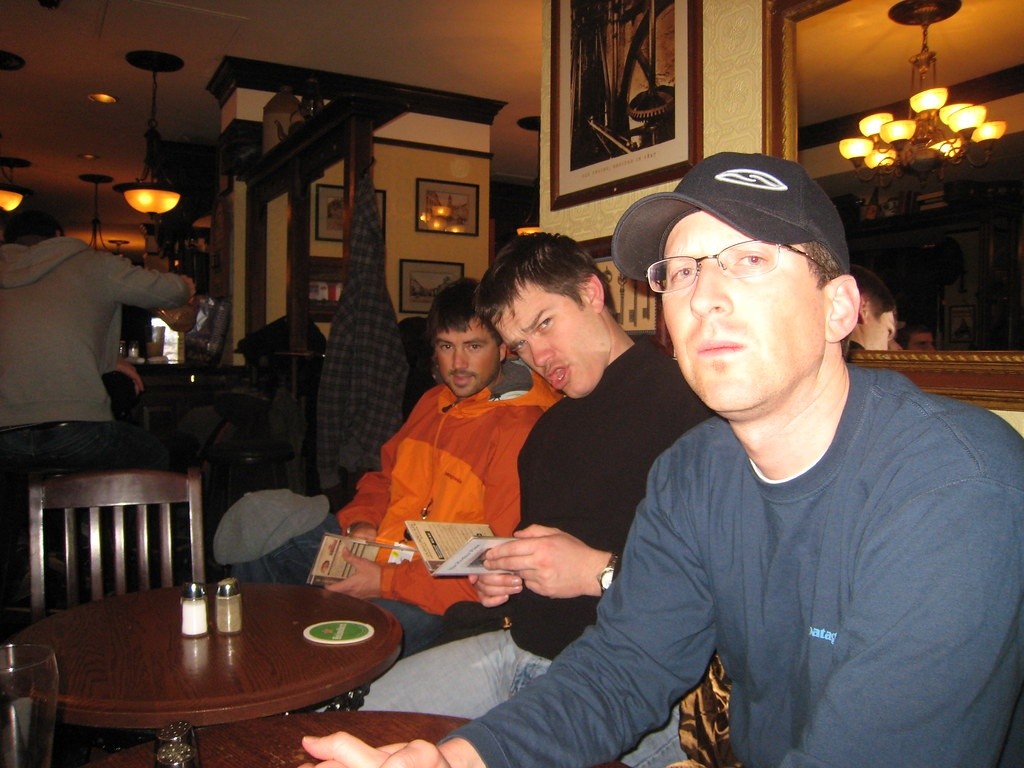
[0,583,404,728]
[85,710,634,768]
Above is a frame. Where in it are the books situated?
[404,520,521,577]
[308,532,416,586]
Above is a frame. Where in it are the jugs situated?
[153,296,197,332]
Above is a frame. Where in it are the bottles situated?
[118,340,126,359]
[153,721,199,768]
[180,582,208,637]
[215,577,242,635]
[127,340,139,360]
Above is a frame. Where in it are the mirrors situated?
[760,0,1024,410]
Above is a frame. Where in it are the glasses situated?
[645,240,830,294]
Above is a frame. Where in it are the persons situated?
[845,262,936,355]
[0,207,197,615]
[297,150,1024,767]
[314,232,725,768]
[224,277,569,667]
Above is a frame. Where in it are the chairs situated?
[25,469,205,626]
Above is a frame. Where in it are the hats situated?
[213,488,331,564]
[611,152,850,281]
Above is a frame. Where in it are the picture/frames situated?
[949,305,976,343]
[414,178,480,237]
[399,258,464,314]
[549,0,704,211]
[315,183,387,244]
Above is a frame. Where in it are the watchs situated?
[596,551,619,593]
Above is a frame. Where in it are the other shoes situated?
[9,550,31,607]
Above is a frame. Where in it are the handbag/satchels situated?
[174,389,307,454]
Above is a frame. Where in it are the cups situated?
[0,643,59,768]
[146,327,165,357]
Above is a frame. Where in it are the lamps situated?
[0,157,29,212]
[110,50,186,220]
[836,0,1005,192]
[516,116,539,236]
